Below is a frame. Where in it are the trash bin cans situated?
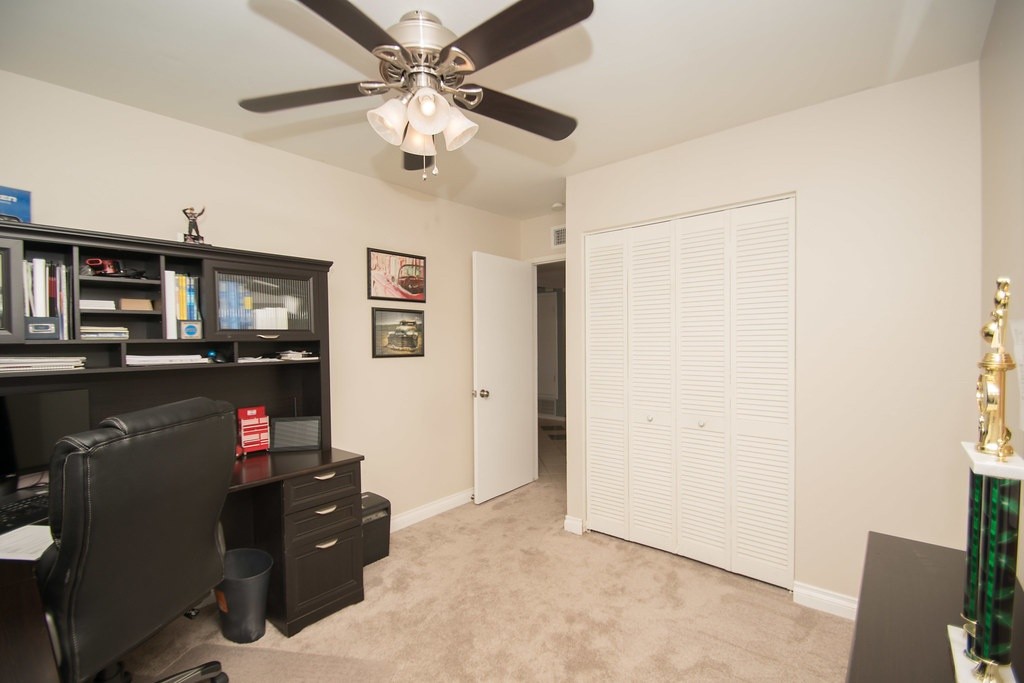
[214,548,274,644]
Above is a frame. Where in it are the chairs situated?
[32,397,238,683]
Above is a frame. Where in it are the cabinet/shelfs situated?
[0,220,333,454]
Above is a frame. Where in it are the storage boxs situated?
[176,309,202,339]
[237,405,270,449]
[264,307,277,329]
[115,298,154,311]
[276,307,288,329]
[24,316,60,340]
[79,299,116,310]
[253,309,267,329]
[0,186,31,223]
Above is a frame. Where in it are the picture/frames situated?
[371,307,425,358]
[367,247,427,303]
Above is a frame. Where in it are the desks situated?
[0,446,366,638]
[844,530,1024,683]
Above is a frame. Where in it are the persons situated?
[183,207,205,244]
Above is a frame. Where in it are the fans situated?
[238,0,596,143]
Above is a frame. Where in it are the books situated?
[21,257,319,365]
[0,357,86,373]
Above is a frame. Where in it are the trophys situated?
[976,278,1016,462]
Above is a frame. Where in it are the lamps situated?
[357,44,485,182]
